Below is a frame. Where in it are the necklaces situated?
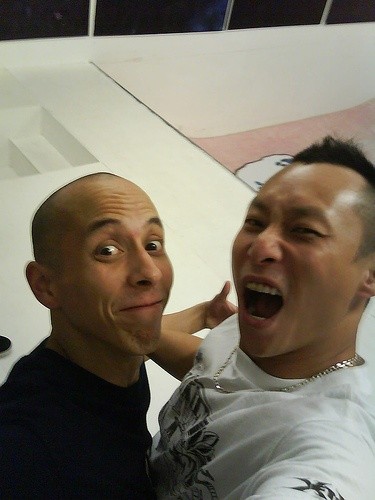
[211,346,364,391]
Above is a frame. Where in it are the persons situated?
[0,173,239,499]
[145,134,375,500]
